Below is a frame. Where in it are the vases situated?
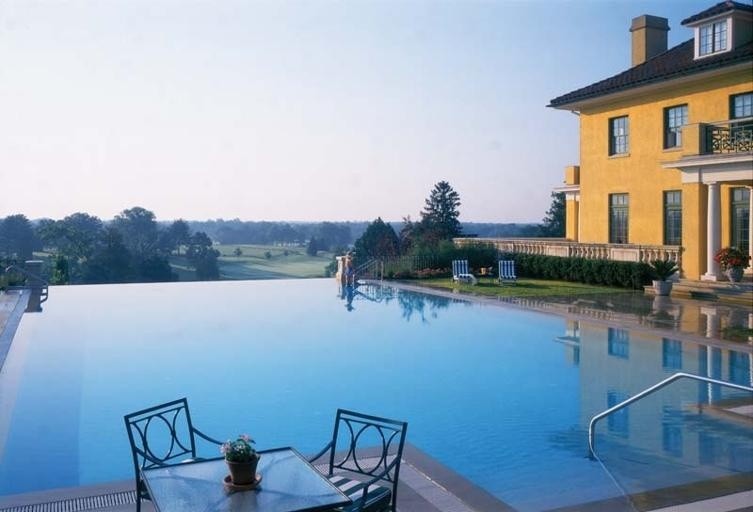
[723,265,743,282]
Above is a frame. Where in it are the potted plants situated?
[644,259,680,296]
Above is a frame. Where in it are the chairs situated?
[123,397,407,511]
[450,258,517,289]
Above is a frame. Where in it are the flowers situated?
[714,248,751,270]
